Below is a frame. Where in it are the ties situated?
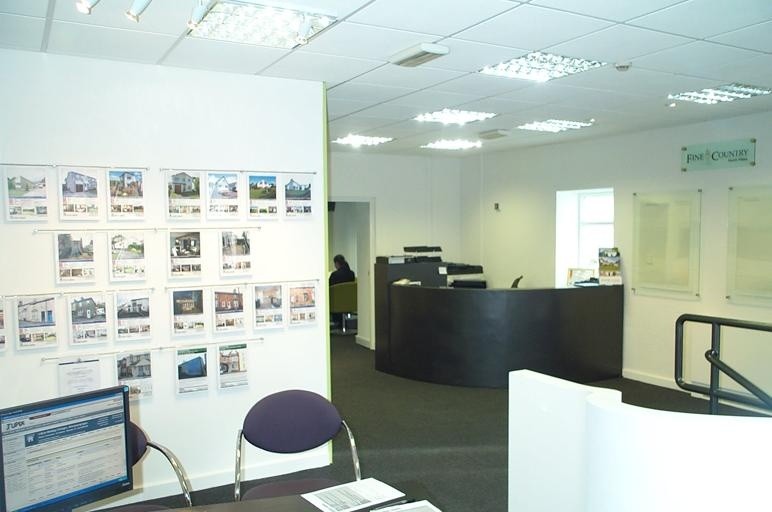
[0,384,134,512]
[453,278,486,288]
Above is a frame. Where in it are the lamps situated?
[330,277,357,336]
[129,389,362,512]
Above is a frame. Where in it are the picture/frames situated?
[150,480,445,512]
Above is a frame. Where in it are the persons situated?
[329,254,355,331]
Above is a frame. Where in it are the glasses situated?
[388,41,450,69]
[75,1,207,29]
